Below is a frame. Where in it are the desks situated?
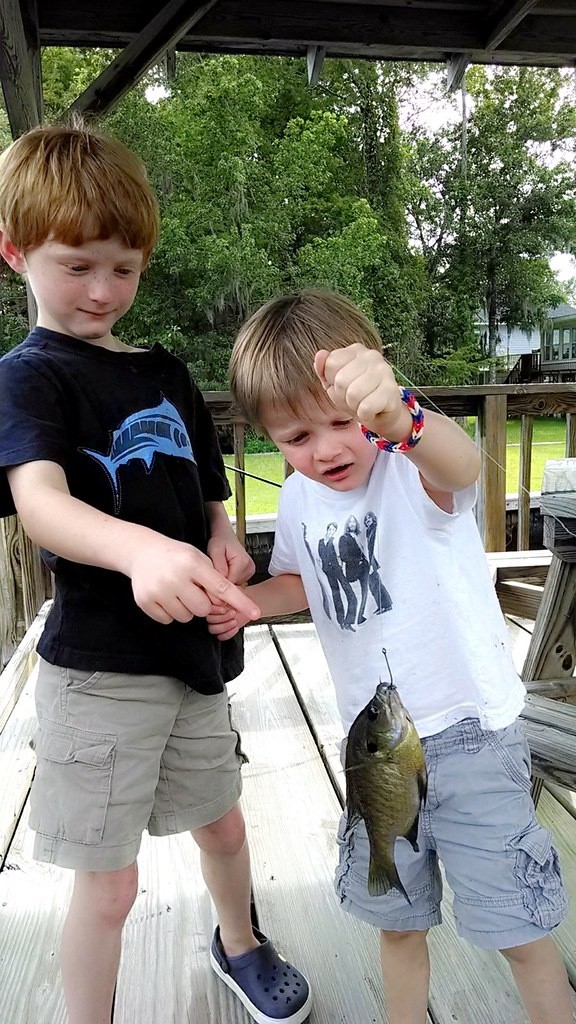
[505,492,576,810]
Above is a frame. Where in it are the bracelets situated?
[361,388,423,453]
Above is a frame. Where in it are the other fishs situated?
[343,683,428,908]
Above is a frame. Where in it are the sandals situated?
[210,923,312,1024]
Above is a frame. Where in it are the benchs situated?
[484,550,555,622]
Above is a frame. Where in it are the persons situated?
[0,127,312,1024]
[302,511,393,632]
[207,291,576,1024]
